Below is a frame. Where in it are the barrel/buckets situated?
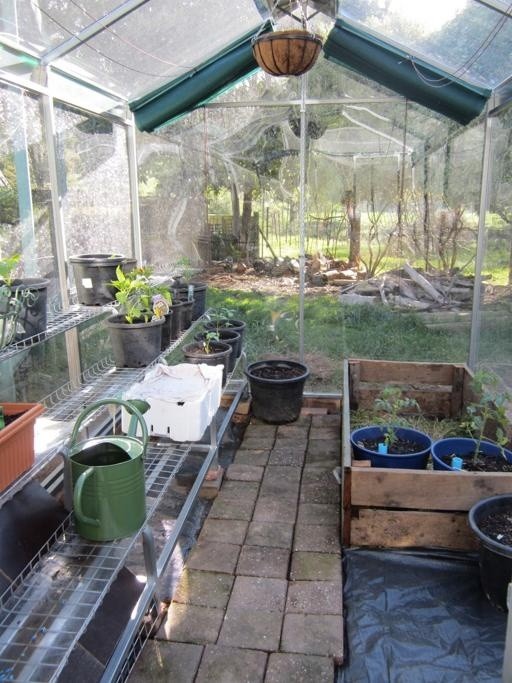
[54,393,155,547]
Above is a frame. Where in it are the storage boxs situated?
[120,362,226,443]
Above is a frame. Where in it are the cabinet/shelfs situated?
[1,273,254,681]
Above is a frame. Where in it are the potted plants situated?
[68,252,126,307]
[202,305,246,359]
[192,307,240,372]
[252,12,331,76]
[101,264,167,369]
[0,249,51,347]
[351,380,434,470]
[181,334,234,390]
[171,252,208,321]
[428,363,511,472]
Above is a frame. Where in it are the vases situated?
[242,358,311,425]
[1,400,46,495]
[117,256,138,282]
[122,264,172,345]
[171,299,183,341]
[173,293,196,331]
[467,490,511,611]
